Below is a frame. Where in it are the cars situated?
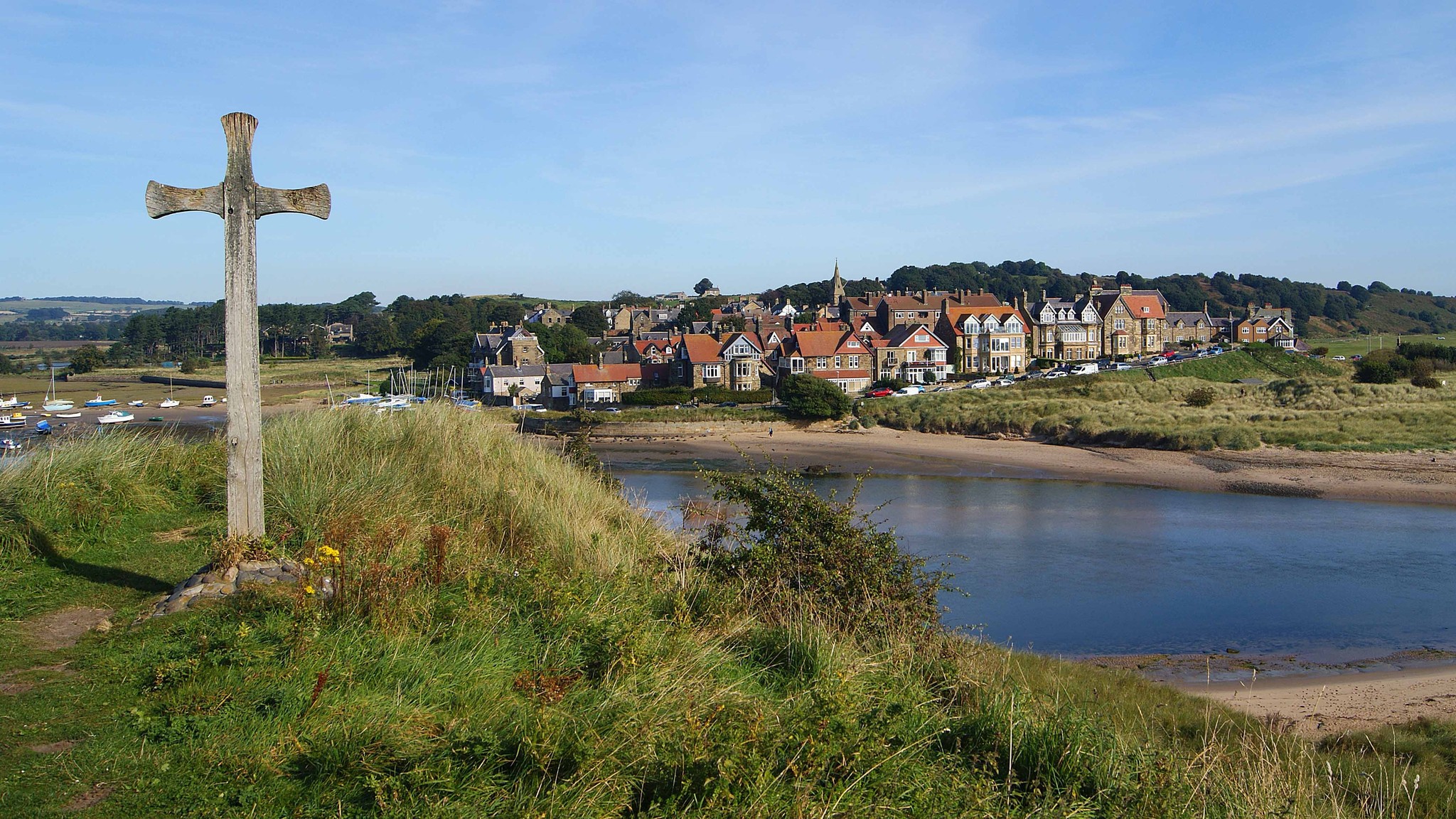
[1146,349,1175,367]
[965,378,992,389]
[1332,355,1346,361]
[1285,349,1326,359]
[712,402,739,408]
[1349,354,1363,362]
[1192,349,1208,358]
[585,407,597,412]
[929,386,954,393]
[1436,336,1446,340]
[994,358,1111,387]
[1208,346,1223,356]
[600,407,621,414]
[1167,354,1184,363]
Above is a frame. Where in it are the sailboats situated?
[325,360,484,415]
[159,374,180,408]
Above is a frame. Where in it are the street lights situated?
[837,371,840,391]
[1228,318,1234,351]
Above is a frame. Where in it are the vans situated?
[510,405,523,411]
[638,405,655,411]
[522,404,547,413]
[891,385,926,397]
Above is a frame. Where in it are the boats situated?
[201,395,218,408]
[148,416,164,422]
[0,365,149,450]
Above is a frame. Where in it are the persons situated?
[1192,342,1194,351]
[1065,363,1069,369]
[276,379,278,384]
[280,379,283,383]
[1059,362,1063,368]
[273,379,276,384]
[942,383,944,385]
[1138,353,1141,362]
[767,427,773,437]
[1131,353,1136,362]
[1195,342,1199,350]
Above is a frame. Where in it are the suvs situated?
[865,387,894,399]
[1109,362,1133,372]
